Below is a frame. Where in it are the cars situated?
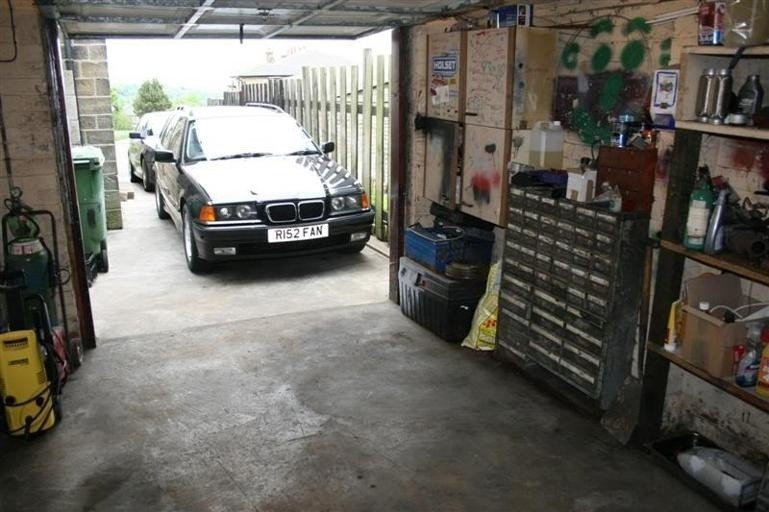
[126,110,175,192]
[153,102,376,273]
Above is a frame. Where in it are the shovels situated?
[598,238,660,446]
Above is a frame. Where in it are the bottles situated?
[694,67,733,125]
[735,327,764,388]
[682,180,731,256]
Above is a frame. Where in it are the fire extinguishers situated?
[683,165,714,251]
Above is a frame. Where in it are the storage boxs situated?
[464,235,494,265]
[397,257,487,343]
[677,274,769,378]
[565,166,597,203]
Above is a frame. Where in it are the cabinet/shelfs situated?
[637,44,769,512]
[424,27,558,229]
[594,144,657,215]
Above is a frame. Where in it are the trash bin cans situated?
[71,145,109,273]
